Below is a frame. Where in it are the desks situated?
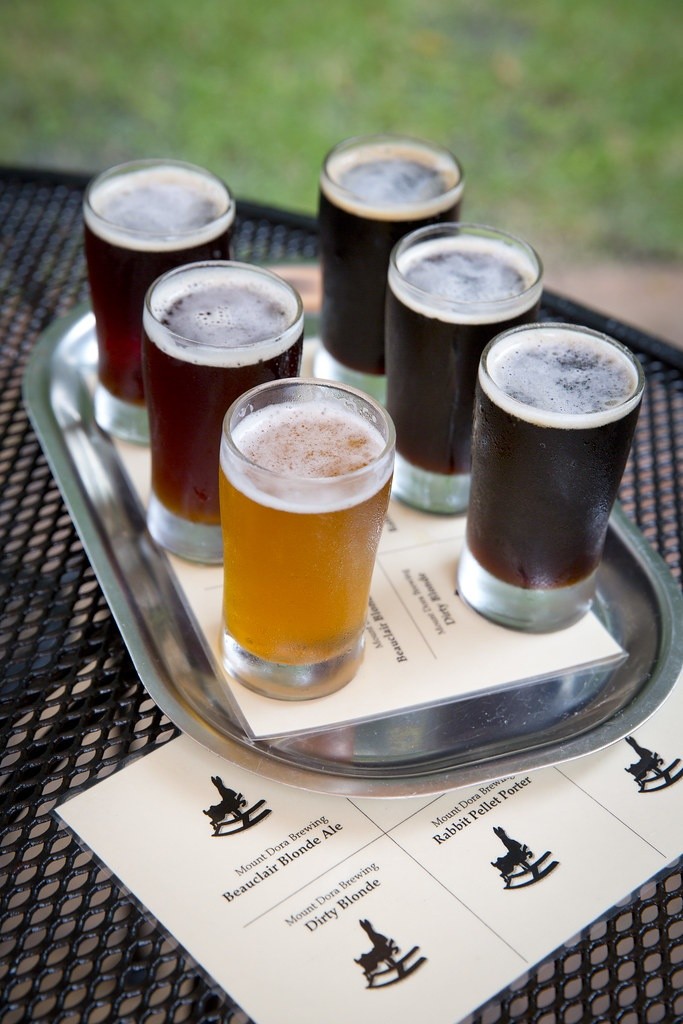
[0,158,683,1024]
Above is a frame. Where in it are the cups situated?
[84,157,236,445]
[319,132,465,375]
[457,323,645,633]
[221,378,396,701]
[143,260,305,567]
[386,223,544,515]
